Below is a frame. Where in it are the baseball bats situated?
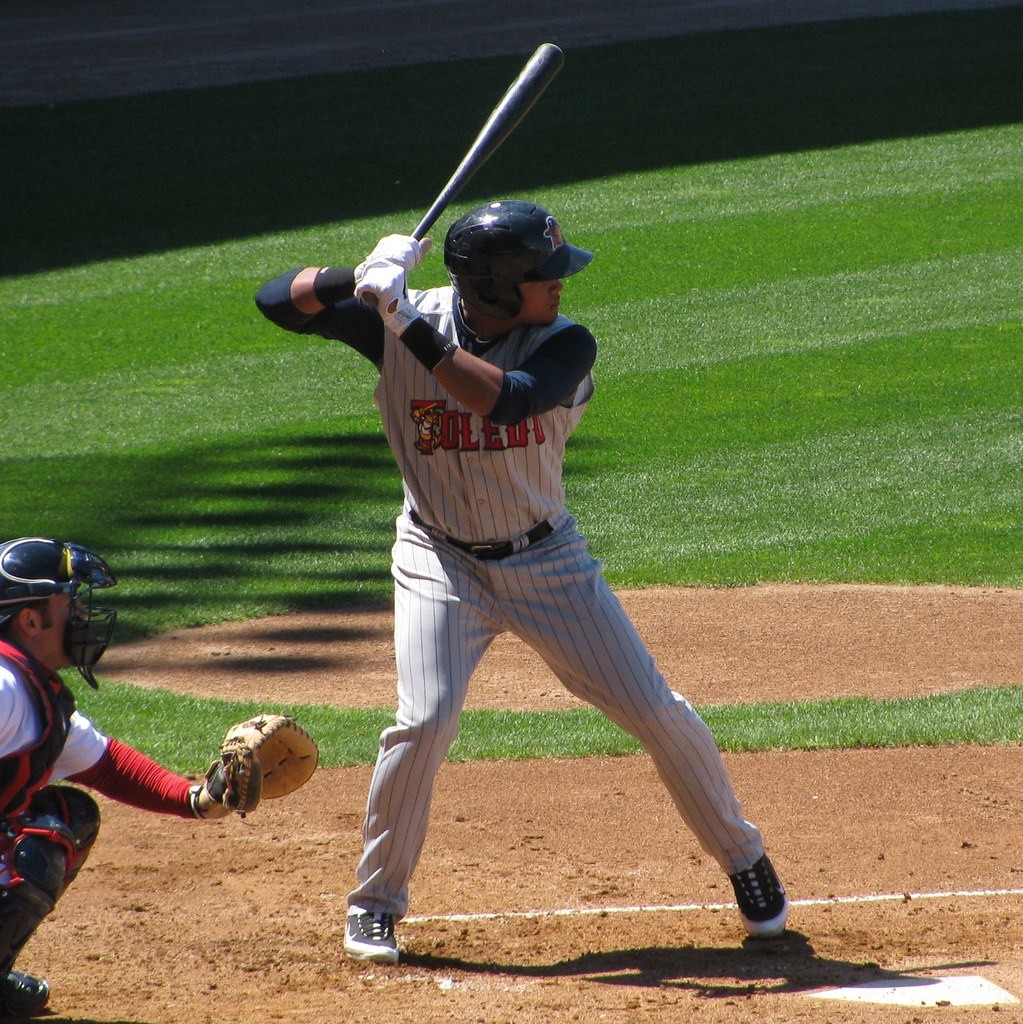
[361,42,564,307]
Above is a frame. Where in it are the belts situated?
[409,509,554,561]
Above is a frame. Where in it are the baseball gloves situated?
[190,714,319,822]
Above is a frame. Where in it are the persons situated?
[0,535,318,1024]
[253,201,788,959]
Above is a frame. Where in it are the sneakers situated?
[344,912,398,963]
[727,852,788,938]
[0,972,49,1014]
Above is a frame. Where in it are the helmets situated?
[1,536,82,623]
[444,200,594,319]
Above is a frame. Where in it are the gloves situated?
[353,234,431,338]
